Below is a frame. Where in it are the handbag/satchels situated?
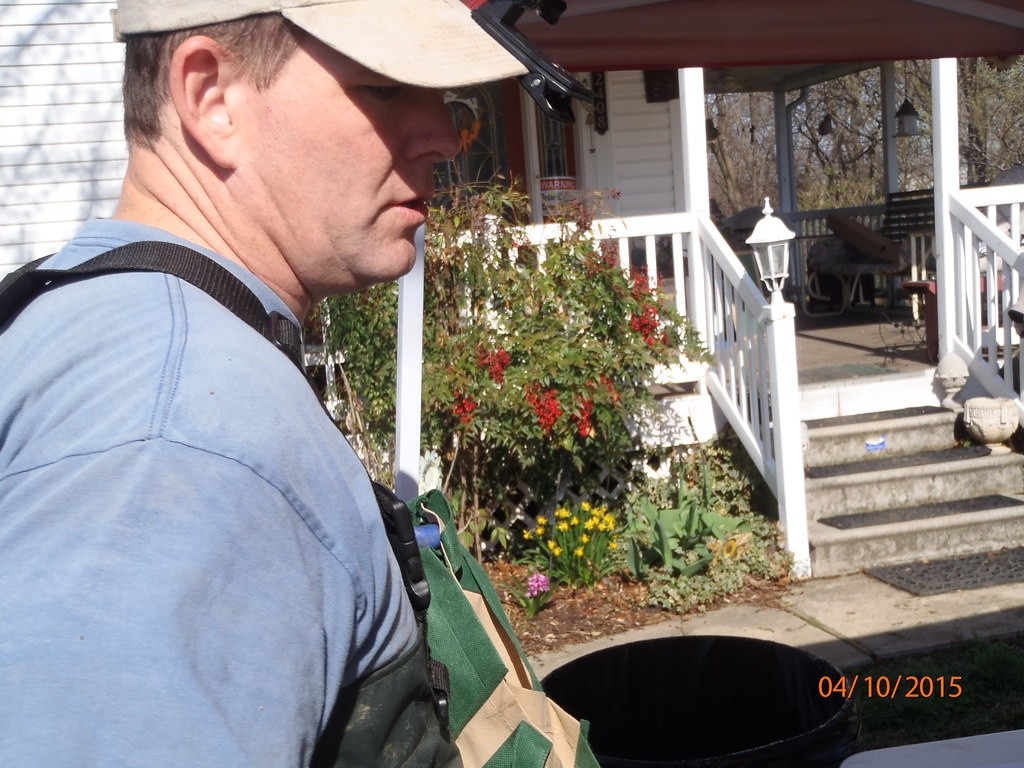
[405,489,601,767]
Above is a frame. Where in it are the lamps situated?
[891,60,922,139]
[744,195,795,305]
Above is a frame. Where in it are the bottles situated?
[537,636,851,768]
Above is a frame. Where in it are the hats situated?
[113,0,530,90]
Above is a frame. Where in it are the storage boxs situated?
[825,215,907,263]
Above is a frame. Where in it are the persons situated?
[0,0,529,768]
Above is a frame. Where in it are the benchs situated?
[903,272,1004,365]
[801,187,935,317]
